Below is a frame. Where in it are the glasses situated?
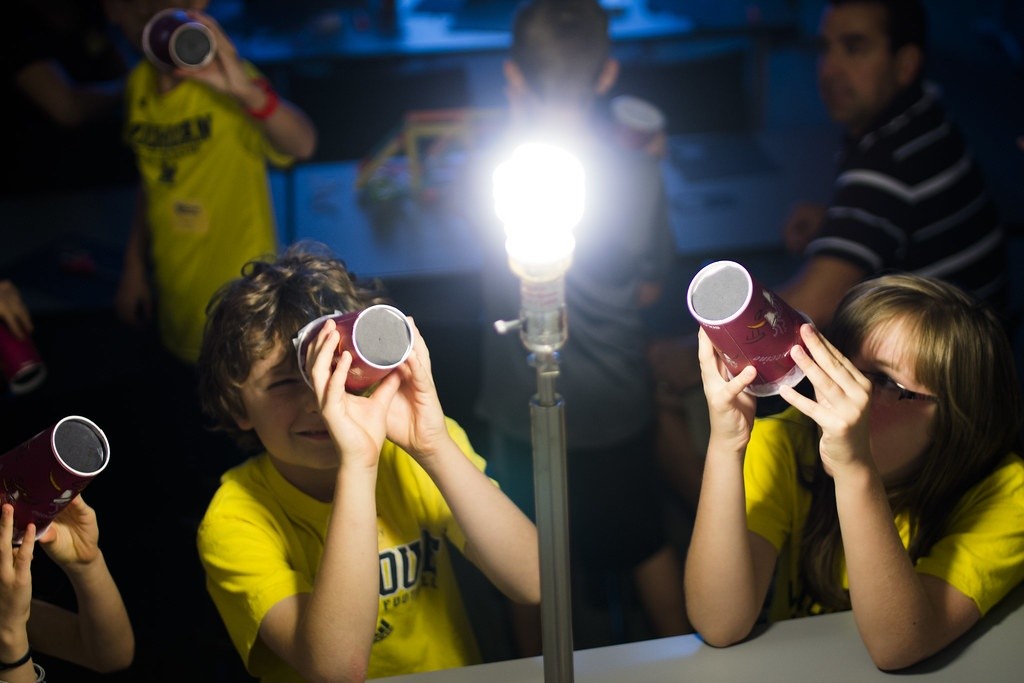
[857,368,941,403]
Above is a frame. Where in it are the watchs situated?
[249,78,277,121]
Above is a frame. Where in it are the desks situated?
[365,595,1024,683]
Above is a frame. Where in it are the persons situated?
[778,0,1005,329]
[682,274,1024,672]
[444,1,696,660]
[197,239,543,682]
[0,491,135,683]
[1,0,317,545]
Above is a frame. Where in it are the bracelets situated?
[0,642,31,670]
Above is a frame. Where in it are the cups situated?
[140,6,218,71]
[297,305,414,391]
[0,330,47,393]
[686,259,818,397]
[0,412,111,544]
[607,96,666,151]
